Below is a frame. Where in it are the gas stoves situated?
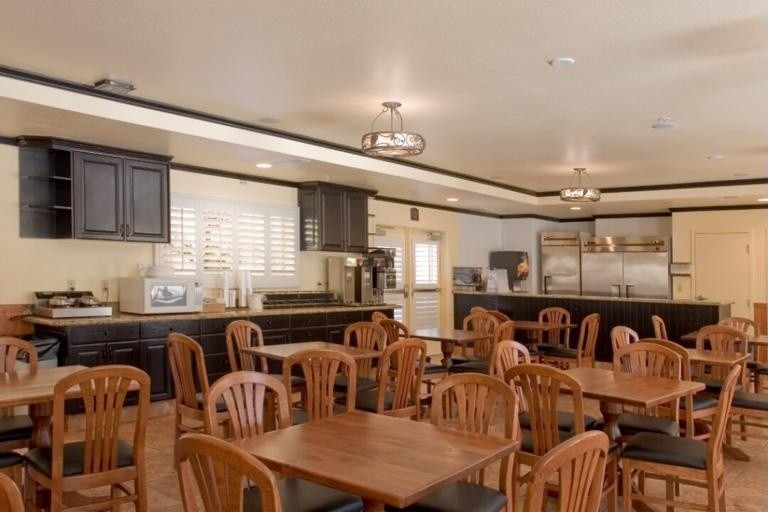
[33,289,113,318]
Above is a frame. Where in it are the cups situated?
[249,295,263,312]
[222,269,253,308]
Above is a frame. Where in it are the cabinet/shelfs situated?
[18,135,71,239]
[71,141,171,244]
[298,181,369,252]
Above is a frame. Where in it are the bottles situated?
[487,265,497,296]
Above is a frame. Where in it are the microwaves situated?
[119,275,204,314]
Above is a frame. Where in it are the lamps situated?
[560,167,602,203]
[361,102,425,158]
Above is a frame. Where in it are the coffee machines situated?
[327,249,397,306]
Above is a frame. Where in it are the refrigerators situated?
[540,230,671,300]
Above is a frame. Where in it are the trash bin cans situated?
[4,333,60,415]
